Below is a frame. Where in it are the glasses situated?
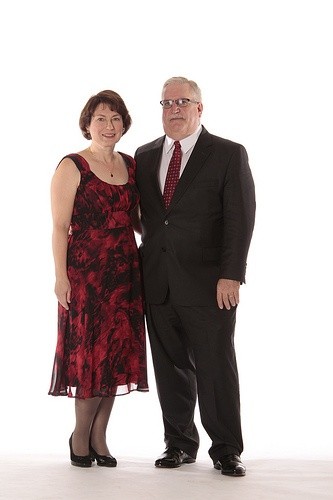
[160,98,199,109]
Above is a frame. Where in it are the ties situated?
[163,140,181,209]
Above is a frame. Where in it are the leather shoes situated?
[155,446,196,467]
[214,454,246,476]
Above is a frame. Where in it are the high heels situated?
[90,435,117,467]
[69,431,91,467]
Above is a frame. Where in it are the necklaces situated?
[110,160,115,177]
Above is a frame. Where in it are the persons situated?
[48,89,150,468]
[133,77,257,478]
